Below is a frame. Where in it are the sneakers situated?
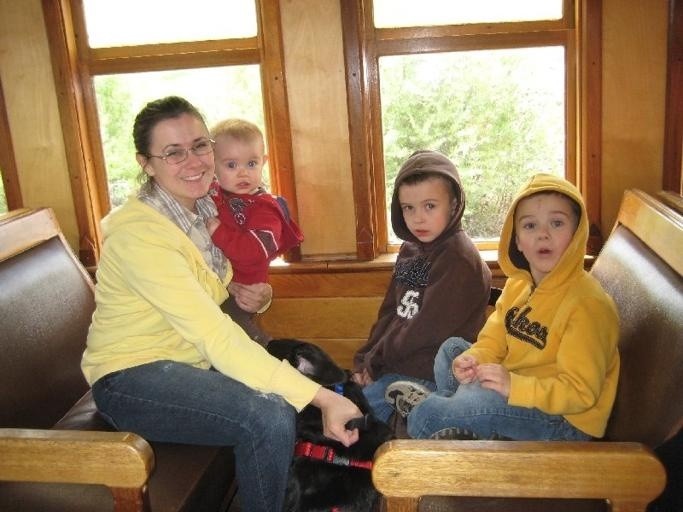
[383,380,431,419]
[427,427,507,440]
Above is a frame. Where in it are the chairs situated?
[370,187,683,511]
[0,198,228,510]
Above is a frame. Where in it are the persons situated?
[346,148,493,442]
[379,172,620,442]
[195,120,304,344]
[77,97,364,511]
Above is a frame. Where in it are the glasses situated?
[141,138,216,165]
